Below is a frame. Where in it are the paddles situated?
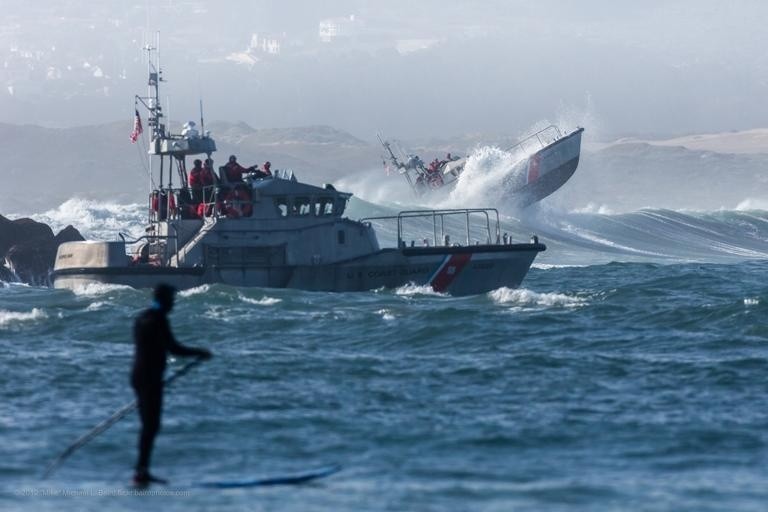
[34,362,204,479]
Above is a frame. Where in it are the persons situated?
[225,155,253,182]
[127,281,215,488]
[261,160,273,180]
[188,159,204,203]
[428,156,439,171]
[199,158,219,207]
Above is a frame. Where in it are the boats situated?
[53,30,547,296]
[375,123,585,211]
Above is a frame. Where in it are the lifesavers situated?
[428,176,441,190]
[224,189,251,215]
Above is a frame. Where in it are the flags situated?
[383,162,390,176]
[130,109,145,145]
